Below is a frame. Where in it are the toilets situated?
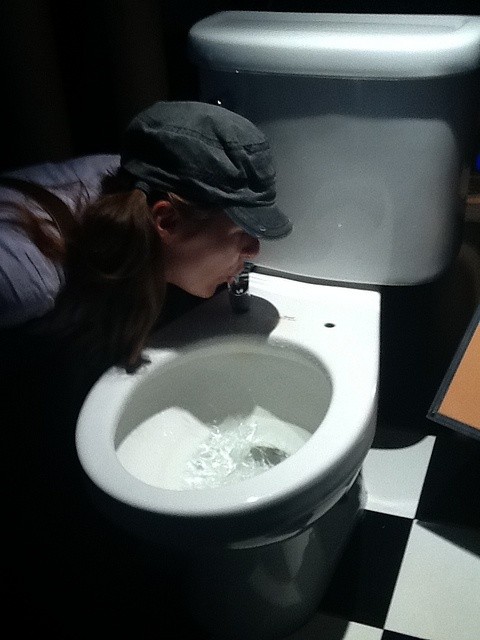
[74,11,480,640]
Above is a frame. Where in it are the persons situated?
[0,100,290,372]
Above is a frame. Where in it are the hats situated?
[119,100,295,241]
